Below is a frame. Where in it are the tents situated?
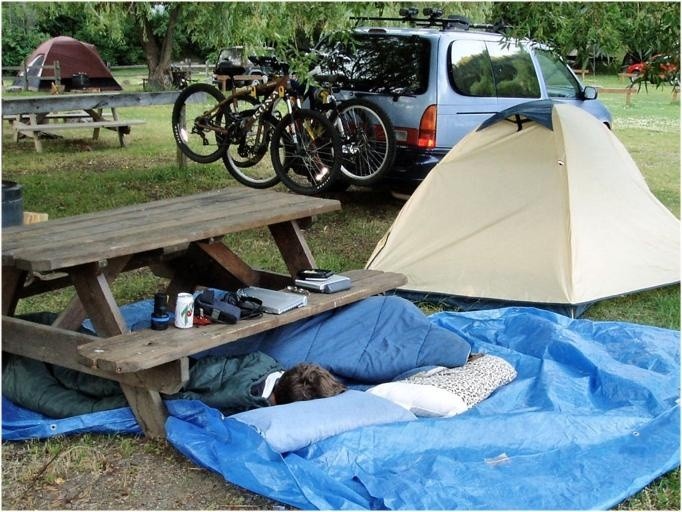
[11,34,125,96]
[360,97,681,328]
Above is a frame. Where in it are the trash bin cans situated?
[2,181,25,226]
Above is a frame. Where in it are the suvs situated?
[297,18,615,206]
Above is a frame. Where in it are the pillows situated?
[231,354,516,462]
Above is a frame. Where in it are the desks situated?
[3,182,340,441]
[1,87,207,153]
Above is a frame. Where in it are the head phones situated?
[225,292,263,319]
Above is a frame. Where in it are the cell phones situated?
[296,268,333,278]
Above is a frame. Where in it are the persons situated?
[494,63,528,98]
[469,61,499,98]
[252,359,348,410]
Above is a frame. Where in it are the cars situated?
[624,52,674,84]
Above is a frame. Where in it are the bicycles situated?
[160,53,402,190]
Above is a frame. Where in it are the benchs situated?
[4,110,145,155]
[572,68,667,104]
[212,72,263,91]
[79,263,407,443]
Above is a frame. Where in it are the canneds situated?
[174,292,195,329]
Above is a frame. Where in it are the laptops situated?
[237,285,307,315]
